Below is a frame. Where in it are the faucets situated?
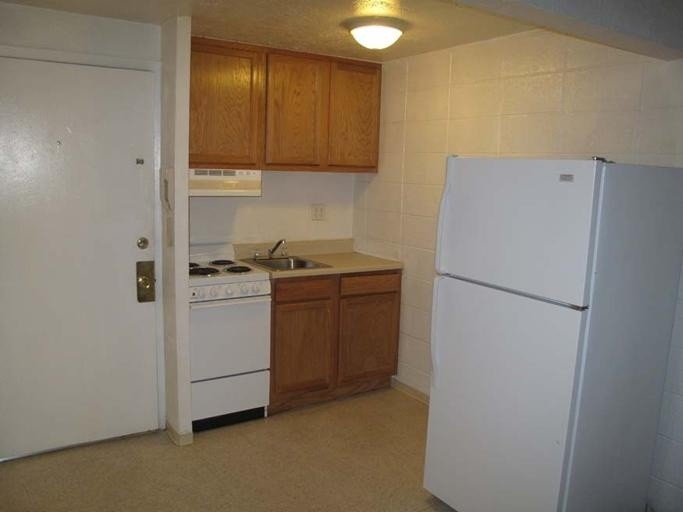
[267,238,288,258]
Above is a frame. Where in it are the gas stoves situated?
[188,259,269,278]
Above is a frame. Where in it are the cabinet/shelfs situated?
[189,36,382,172]
[269,269,402,416]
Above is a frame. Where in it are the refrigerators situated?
[419,153,681,511]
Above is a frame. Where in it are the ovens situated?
[188,279,271,383]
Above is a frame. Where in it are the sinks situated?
[240,254,333,272]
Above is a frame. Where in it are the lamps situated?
[339,16,410,50]
